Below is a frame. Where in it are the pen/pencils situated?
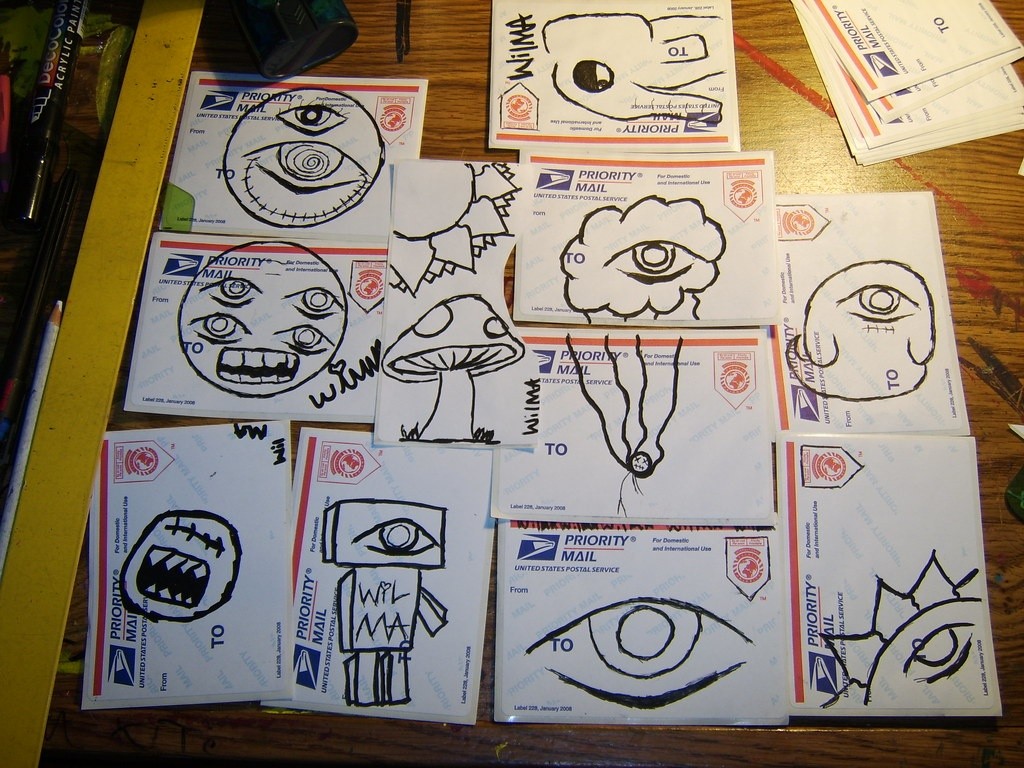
[12,0,93,221]
[0,167,86,407]
[3,294,67,580]
